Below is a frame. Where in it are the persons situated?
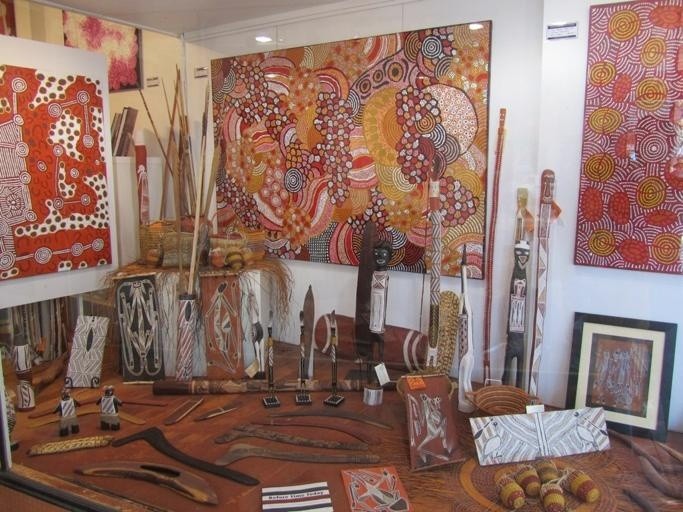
[369,237,394,363]
[243,287,263,373]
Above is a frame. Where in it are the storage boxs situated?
[111,250,280,384]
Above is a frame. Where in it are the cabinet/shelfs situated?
[66,156,163,345]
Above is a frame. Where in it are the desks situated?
[1,342,683,510]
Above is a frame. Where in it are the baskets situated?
[465,385,541,415]
[138,220,194,267]
[208,207,246,249]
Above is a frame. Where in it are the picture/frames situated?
[566,312,677,443]
